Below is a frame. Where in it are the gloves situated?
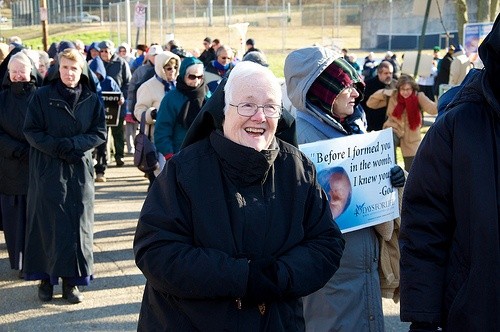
[57,141,74,158]
[228,243,280,294]
[390,166,405,186]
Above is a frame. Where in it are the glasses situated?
[165,66,177,70]
[228,103,282,118]
[187,74,204,80]
[220,55,232,60]
[340,83,358,94]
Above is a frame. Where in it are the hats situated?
[98,40,114,49]
[454,45,464,53]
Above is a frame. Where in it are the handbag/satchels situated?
[133,134,158,172]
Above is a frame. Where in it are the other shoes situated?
[39,279,53,300]
[63,289,80,303]
[116,160,123,166]
[95,172,106,182]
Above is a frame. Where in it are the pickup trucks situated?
[62,12,101,24]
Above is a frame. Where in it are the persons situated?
[431,43,472,97]
[133,60,345,332]
[0,34,50,271]
[398,14,500,332]
[23,48,108,304]
[46,38,269,189]
[342,47,439,170]
[284,48,405,332]
